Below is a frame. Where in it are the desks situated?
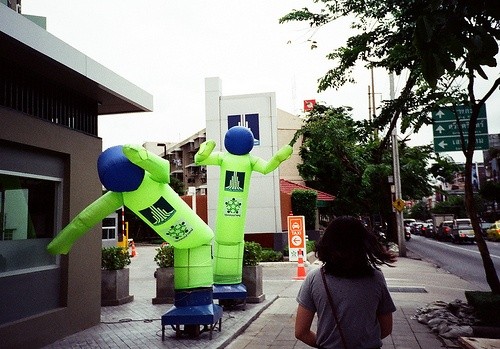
[458,336,500,349]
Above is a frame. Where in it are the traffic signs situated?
[432,103,490,154]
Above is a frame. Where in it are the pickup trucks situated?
[450,219,481,244]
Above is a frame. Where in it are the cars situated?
[435,221,454,240]
[481,222,500,241]
[403,214,433,237]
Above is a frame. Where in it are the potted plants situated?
[152,246,175,305]
[242,241,265,303]
[101,245,133,305]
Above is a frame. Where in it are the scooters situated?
[404,228,411,240]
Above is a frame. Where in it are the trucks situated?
[433,213,455,239]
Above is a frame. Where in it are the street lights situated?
[367,85,382,141]
[157,143,167,159]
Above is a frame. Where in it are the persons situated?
[294,214,397,349]
[404,223,412,237]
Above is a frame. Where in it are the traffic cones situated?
[293,249,307,280]
